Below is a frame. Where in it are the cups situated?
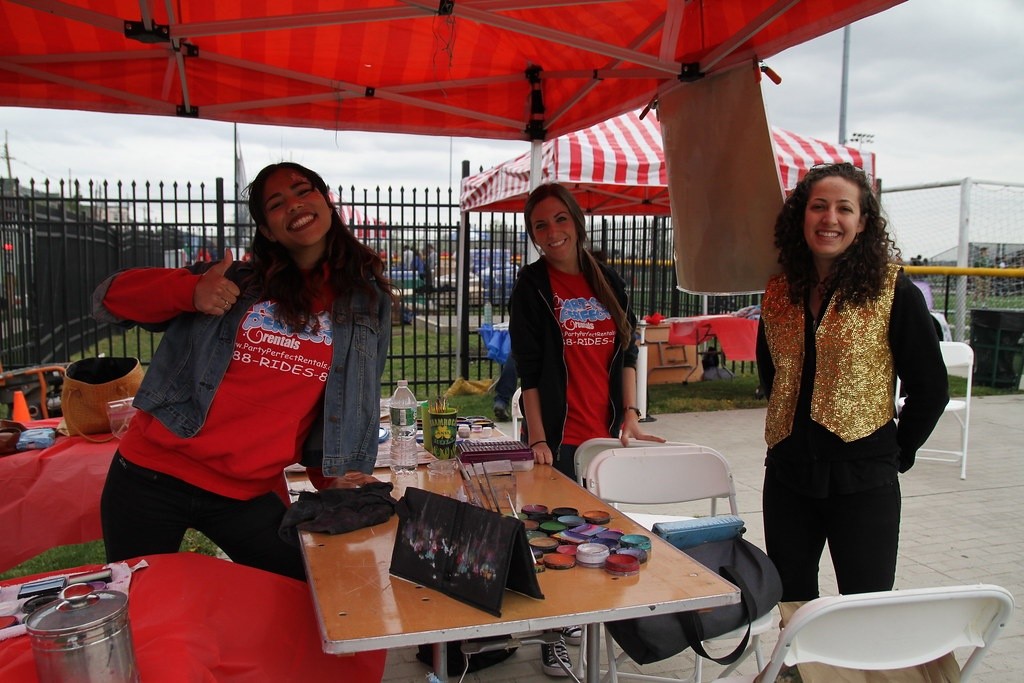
[428,407,458,460]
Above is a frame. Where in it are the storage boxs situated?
[456,441,534,472]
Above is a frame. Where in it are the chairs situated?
[512,387,524,441]
[753,584,1015,683]
[585,445,763,683]
[573,438,717,683]
[893,341,975,479]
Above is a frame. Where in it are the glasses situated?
[810,163,863,173]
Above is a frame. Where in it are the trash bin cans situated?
[967,308,1024,392]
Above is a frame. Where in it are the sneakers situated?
[542,635,572,677]
[543,624,581,645]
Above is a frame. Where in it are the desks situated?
[0,552,389,683]
[412,315,513,335]
[0,409,137,574]
[667,315,759,385]
[634,321,706,385]
[281,400,742,683]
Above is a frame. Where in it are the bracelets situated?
[626,407,642,421]
[530,441,547,449]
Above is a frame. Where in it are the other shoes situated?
[494,402,510,420]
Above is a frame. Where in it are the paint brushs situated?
[482,461,503,515]
[470,459,495,513]
[454,455,485,510]
[428,397,449,412]
[505,490,538,565]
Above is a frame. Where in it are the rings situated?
[221,301,228,308]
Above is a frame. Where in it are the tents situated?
[327,189,390,284]
[455,102,877,379]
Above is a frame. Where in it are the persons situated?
[493,348,519,421]
[756,164,951,601]
[381,242,439,287]
[909,255,930,283]
[970,247,990,307]
[508,183,667,676]
[92,162,393,581]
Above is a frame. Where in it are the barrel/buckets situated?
[970,309,1024,388]
[25,585,142,683]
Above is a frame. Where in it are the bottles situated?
[484,301,492,324]
[389,380,418,474]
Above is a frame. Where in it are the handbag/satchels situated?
[602,535,783,665]
[60,357,143,442]
